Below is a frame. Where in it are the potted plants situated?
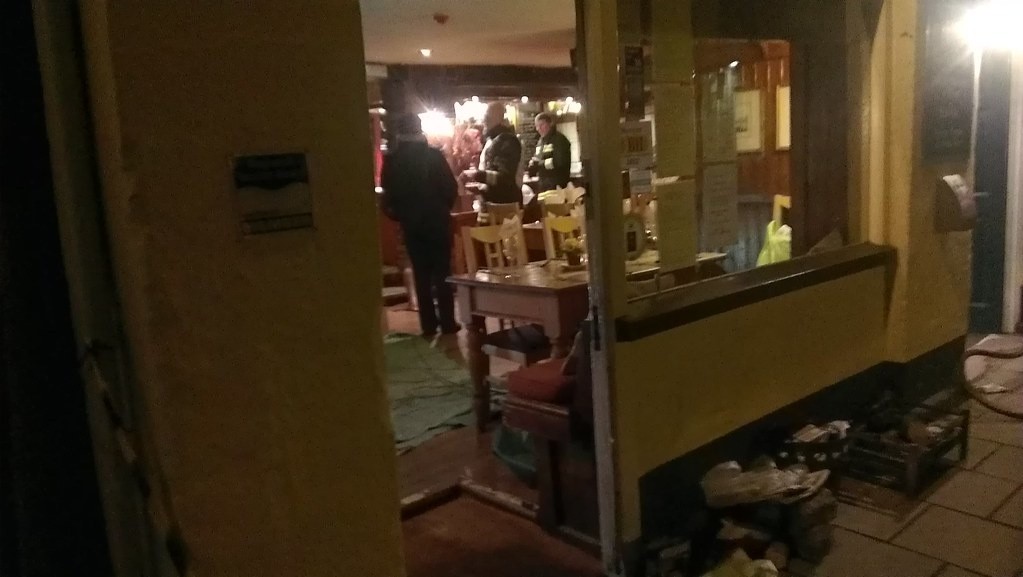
[565,253,581,265]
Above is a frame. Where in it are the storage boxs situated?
[839,396,969,498]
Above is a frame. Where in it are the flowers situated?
[560,238,585,257]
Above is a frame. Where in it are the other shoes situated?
[443,322,462,334]
[423,323,437,333]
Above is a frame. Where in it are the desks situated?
[445,250,727,433]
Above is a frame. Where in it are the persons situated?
[380,111,462,335]
[529,114,572,195]
[460,102,528,264]
[435,116,484,176]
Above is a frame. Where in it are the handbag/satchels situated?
[380,154,400,220]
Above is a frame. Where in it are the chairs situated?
[452,199,649,531]
